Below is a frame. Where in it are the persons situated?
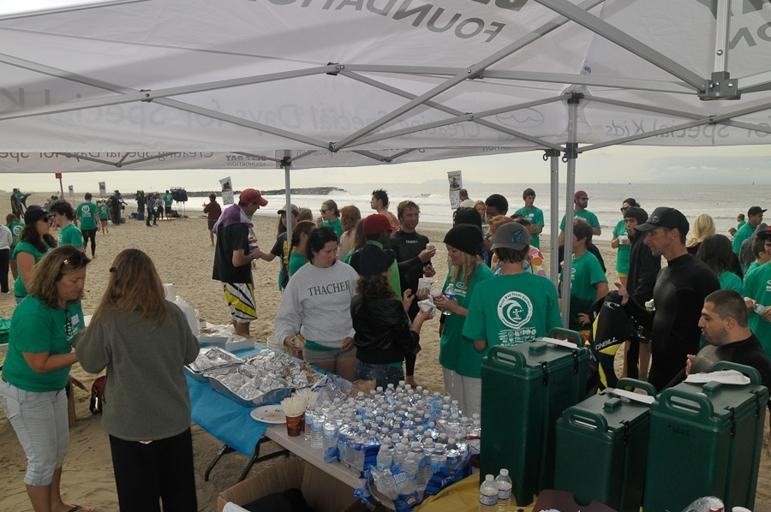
[74,249,200,512]
[0,181,198,305]
[202,182,545,390]
[433,187,771,468]
[1,245,90,512]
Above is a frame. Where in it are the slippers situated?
[68,504,95,512]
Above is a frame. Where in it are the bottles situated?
[301,380,479,494]
[747,300,769,319]
[495,469,511,511]
[439,283,456,317]
[479,474,497,511]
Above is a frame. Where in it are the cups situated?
[286,409,310,436]
[419,278,433,293]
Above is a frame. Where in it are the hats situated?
[240,189,267,206]
[362,214,396,234]
[444,223,484,256]
[278,204,299,214]
[349,244,395,280]
[489,222,531,251]
[24,205,48,224]
[633,207,689,231]
[748,207,767,217]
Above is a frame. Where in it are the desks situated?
[182,341,353,487]
[65,348,107,423]
[263,402,480,512]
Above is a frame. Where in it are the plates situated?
[248,405,289,425]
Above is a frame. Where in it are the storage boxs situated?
[217,457,364,512]
[479,338,590,507]
[554,388,651,512]
[643,372,768,512]
